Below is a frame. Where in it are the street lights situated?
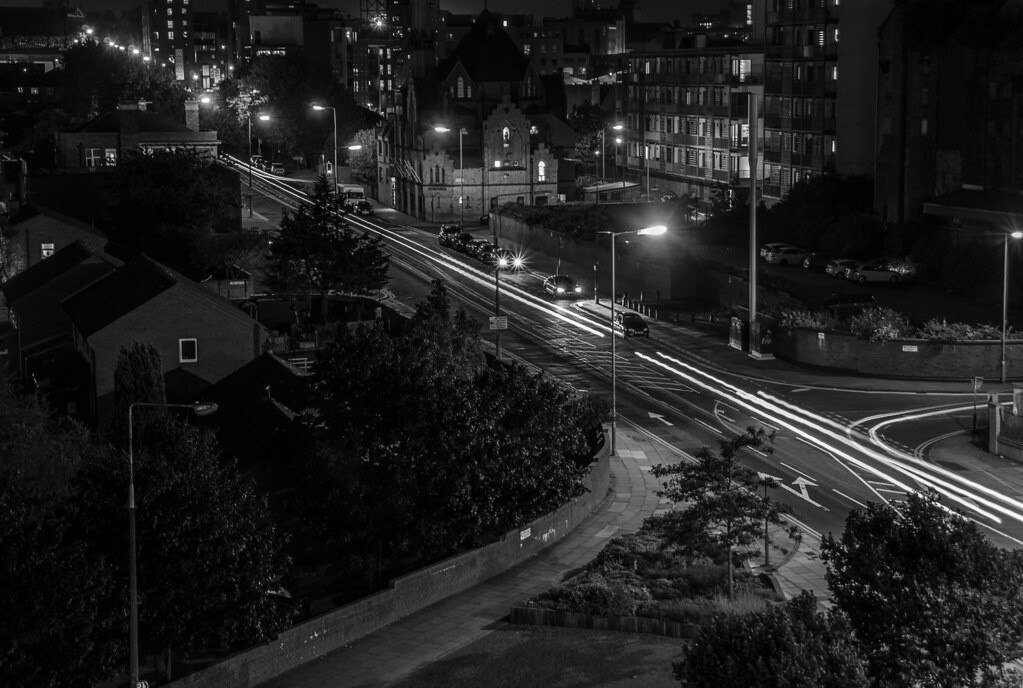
[126,402,218,688]
[1002,231,1023,382]
[575,224,667,456]
[313,105,362,240]
[602,124,628,202]
[249,115,269,190]
[434,126,467,228]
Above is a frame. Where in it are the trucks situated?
[337,183,366,211]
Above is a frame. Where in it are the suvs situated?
[438,224,464,246]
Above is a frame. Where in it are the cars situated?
[543,274,582,300]
[250,155,286,176]
[450,232,519,271]
[611,312,649,340]
[353,200,374,216]
